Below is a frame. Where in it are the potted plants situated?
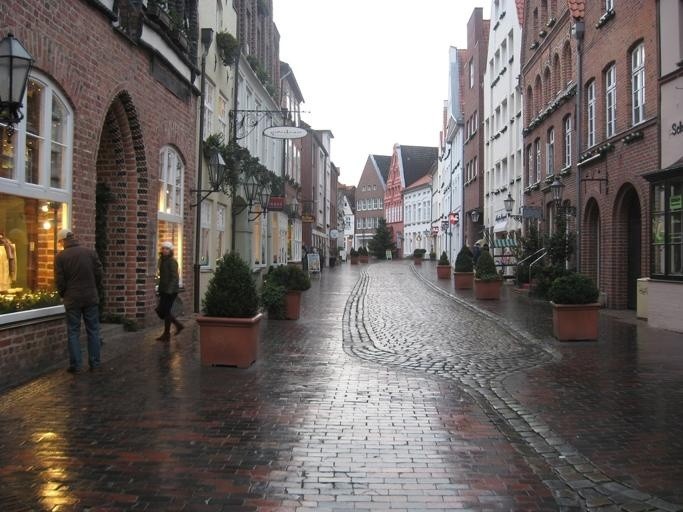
[473,250,504,301]
[436,250,452,280]
[452,244,474,290]
[359,246,368,263]
[413,248,426,266]
[546,272,601,343]
[350,247,359,264]
[260,261,312,321]
[195,246,264,370]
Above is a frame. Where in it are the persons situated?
[53,228,103,372]
[152,240,184,342]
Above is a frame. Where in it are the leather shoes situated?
[155,324,185,342]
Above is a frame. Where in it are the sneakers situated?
[88,367,106,378]
[67,366,81,374]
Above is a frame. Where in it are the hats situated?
[162,241,174,251]
[58,229,72,242]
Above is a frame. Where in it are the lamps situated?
[231,171,259,216]
[0,30,35,146]
[188,150,227,211]
[504,191,522,223]
[248,193,269,222]
[547,172,576,218]
[470,210,480,224]
